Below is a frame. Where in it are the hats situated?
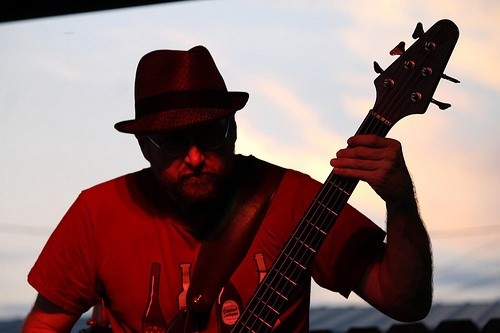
[114,46,249,134]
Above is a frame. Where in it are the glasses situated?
[145,120,228,153]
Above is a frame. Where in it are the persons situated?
[17,47,433,333]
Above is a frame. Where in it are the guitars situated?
[231,17,461,333]
